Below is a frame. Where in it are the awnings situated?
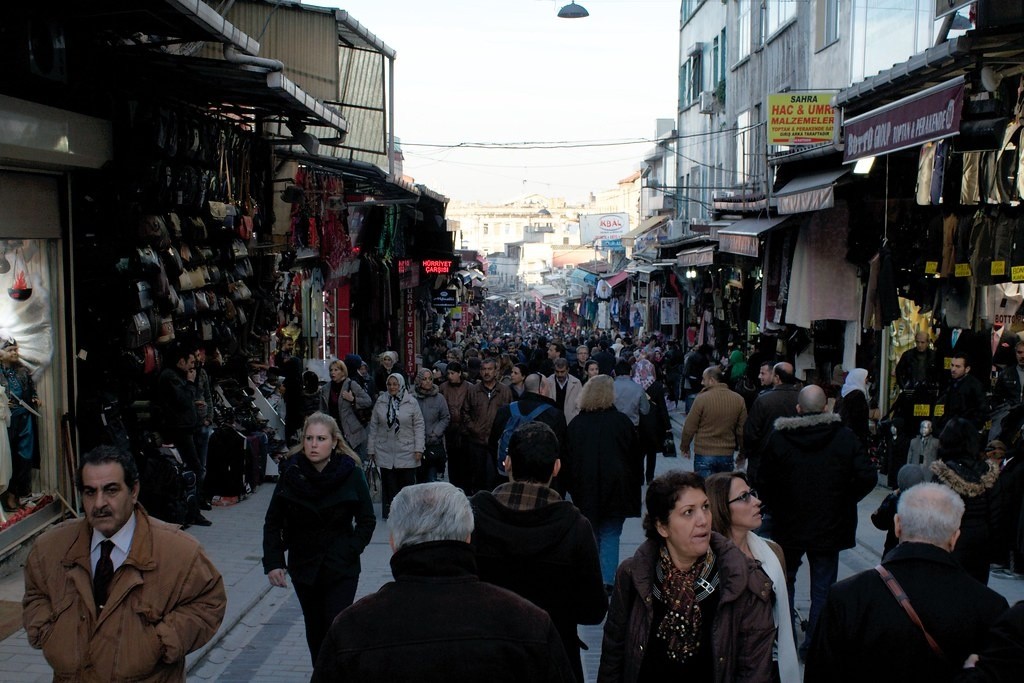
[717,214,791,258]
[676,244,717,267]
[774,169,851,214]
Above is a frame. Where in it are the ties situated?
[952,330,957,348]
[92,540,115,619]
[991,333,998,372]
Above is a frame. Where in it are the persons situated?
[1,336,42,511]
[870,417,1003,586]
[755,384,878,661]
[309,481,575,683]
[262,411,377,670]
[22,445,227,683]
[185,347,214,510]
[803,483,1010,683]
[881,323,1024,575]
[273,300,878,596]
[157,351,212,526]
[597,469,777,683]
[954,601,1024,683]
[702,471,800,683]
[907,420,939,466]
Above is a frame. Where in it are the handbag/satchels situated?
[114,118,282,375]
[662,430,678,458]
[348,380,372,429]
[734,373,755,394]
[363,456,382,498]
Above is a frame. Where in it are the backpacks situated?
[496,399,553,477]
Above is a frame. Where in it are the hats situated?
[382,351,398,365]
[446,347,463,361]
[0,338,17,349]
[433,362,448,376]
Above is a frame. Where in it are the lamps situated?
[291,133,321,156]
[557,0,589,18]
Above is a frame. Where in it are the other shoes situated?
[18,492,45,505]
[6,505,20,511]
[190,515,212,526]
[198,498,212,510]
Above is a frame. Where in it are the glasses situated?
[447,355,455,359]
[386,382,398,386]
[432,367,440,371]
[727,489,759,504]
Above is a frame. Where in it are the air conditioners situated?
[697,90,712,114]
